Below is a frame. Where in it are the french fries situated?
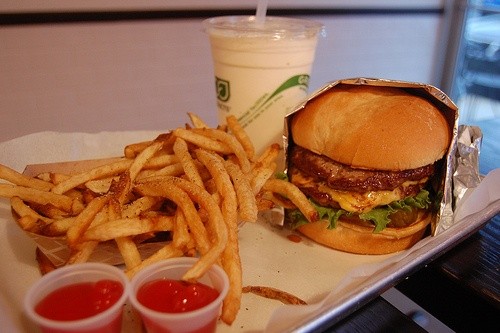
[0,111,319,324]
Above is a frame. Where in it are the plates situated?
[0,176,499,333]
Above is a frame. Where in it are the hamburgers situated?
[286,84,453,255]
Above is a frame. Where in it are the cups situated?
[128,257,229,333]
[199,15,324,171]
[23,262,128,333]
